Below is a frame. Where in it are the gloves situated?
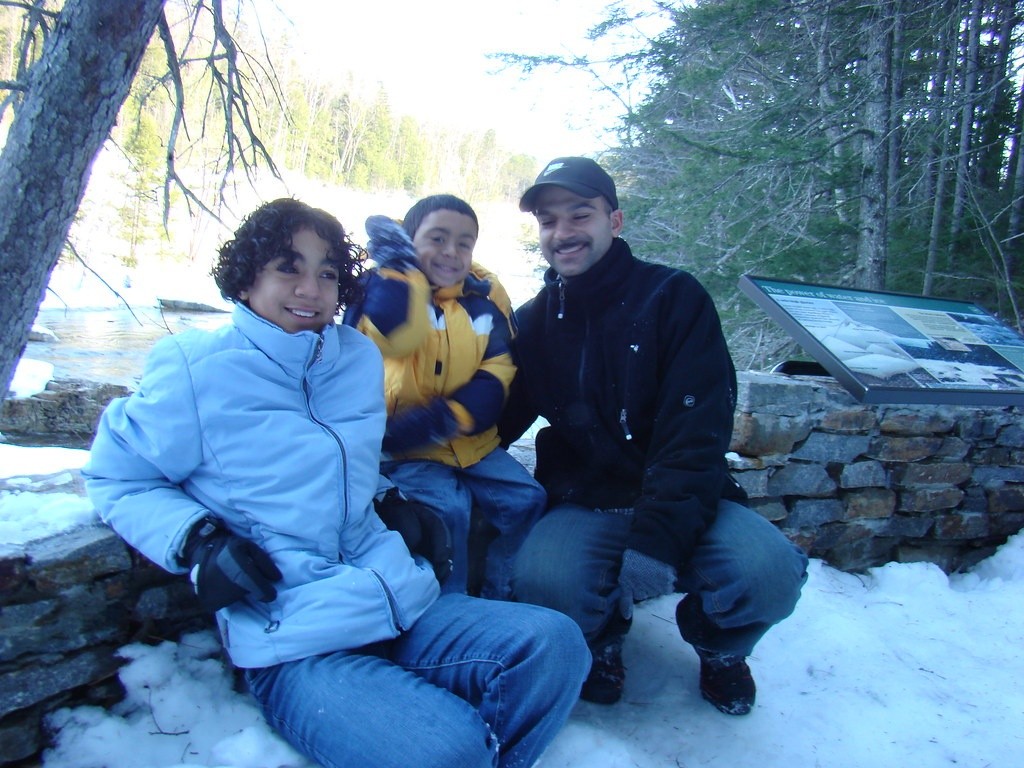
[617,549,677,620]
[365,213,418,267]
[382,399,460,456]
[375,485,453,584]
[176,515,282,612]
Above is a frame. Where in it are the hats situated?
[519,156,618,215]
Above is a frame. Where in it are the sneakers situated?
[674,599,757,715]
[580,628,628,705]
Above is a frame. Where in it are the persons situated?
[496,156,810,715]
[342,193,546,602]
[87,197,591,768]
